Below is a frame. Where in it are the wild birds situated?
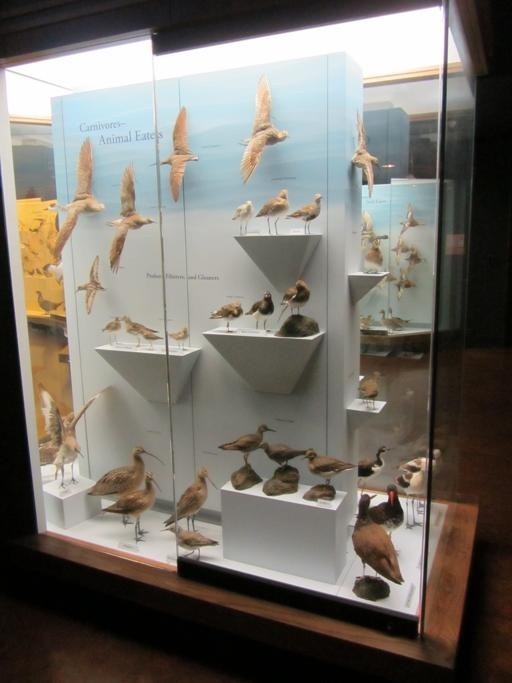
[359,202,422,332]
[87,445,220,561]
[352,446,445,585]
[107,161,157,275]
[232,188,324,236]
[102,315,189,350]
[208,279,310,332]
[219,424,358,492]
[76,256,105,315]
[162,108,200,203]
[50,136,105,261]
[239,73,288,185]
[351,108,383,197]
[37,382,116,488]
